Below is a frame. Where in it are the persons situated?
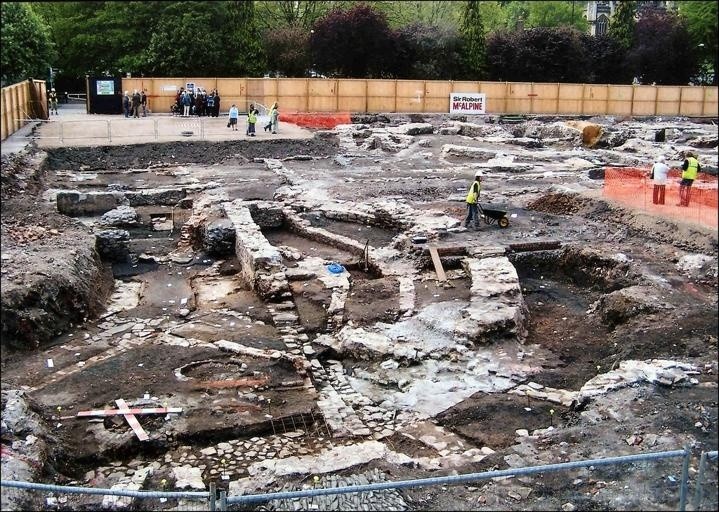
[229,104,239,130]
[246,102,280,136]
[48,88,59,115]
[176,86,220,118]
[650,156,671,205]
[462,170,484,231]
[122,90,147,118]
[675,152,701,206]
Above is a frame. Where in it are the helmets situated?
[476,171,484,177]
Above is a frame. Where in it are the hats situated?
[658,156,665,162]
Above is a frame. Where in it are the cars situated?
[305,69,328,79]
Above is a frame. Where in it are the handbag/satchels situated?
[650,168,654,179]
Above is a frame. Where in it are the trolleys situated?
[476,201,510,228]
